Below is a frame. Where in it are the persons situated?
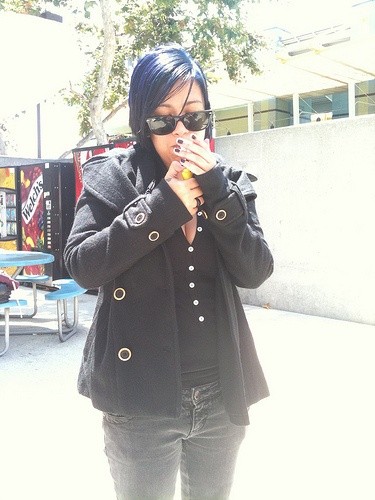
[64,49,274,500]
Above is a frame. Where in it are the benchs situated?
[45,277,91,345]
[0,300,28,357]
[15,275,50,318]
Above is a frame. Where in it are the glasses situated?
[145,110,213,135]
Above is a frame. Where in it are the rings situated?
[195,197,201,207]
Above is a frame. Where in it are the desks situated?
[0,250,55,280]
